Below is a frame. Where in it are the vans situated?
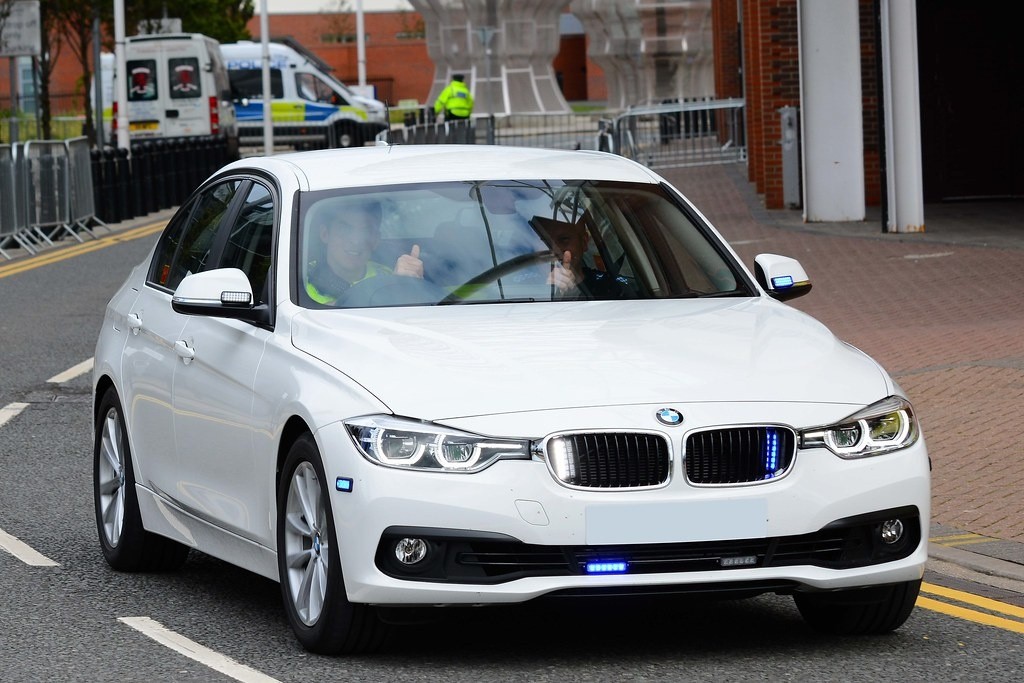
[112,32,238,150]
[101,36,387,147]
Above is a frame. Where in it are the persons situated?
[434,74,474,122]
[306,194,424,306]
[520,203,641,299]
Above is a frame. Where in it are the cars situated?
[90,143,932,655]
